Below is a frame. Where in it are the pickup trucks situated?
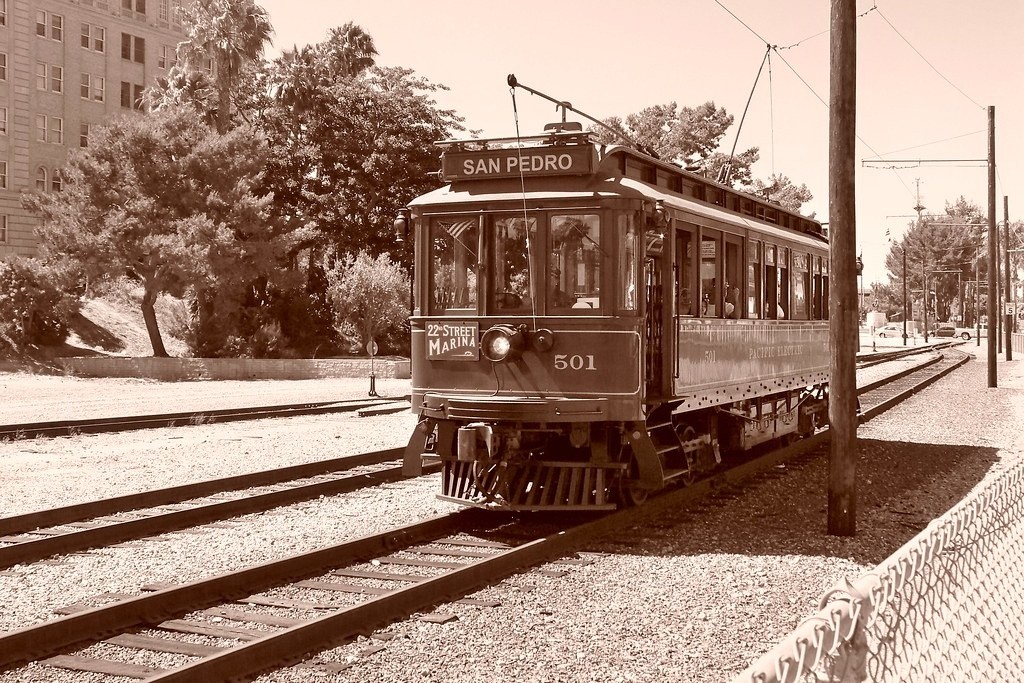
[954,323,989,341]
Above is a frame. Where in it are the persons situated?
[765,294,784,319]
[680,277,741,318]
[550,263,575,308]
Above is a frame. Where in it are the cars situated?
[929,326,955,338]
[875,326,913,338]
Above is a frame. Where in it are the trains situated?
[392,121,862,513]
